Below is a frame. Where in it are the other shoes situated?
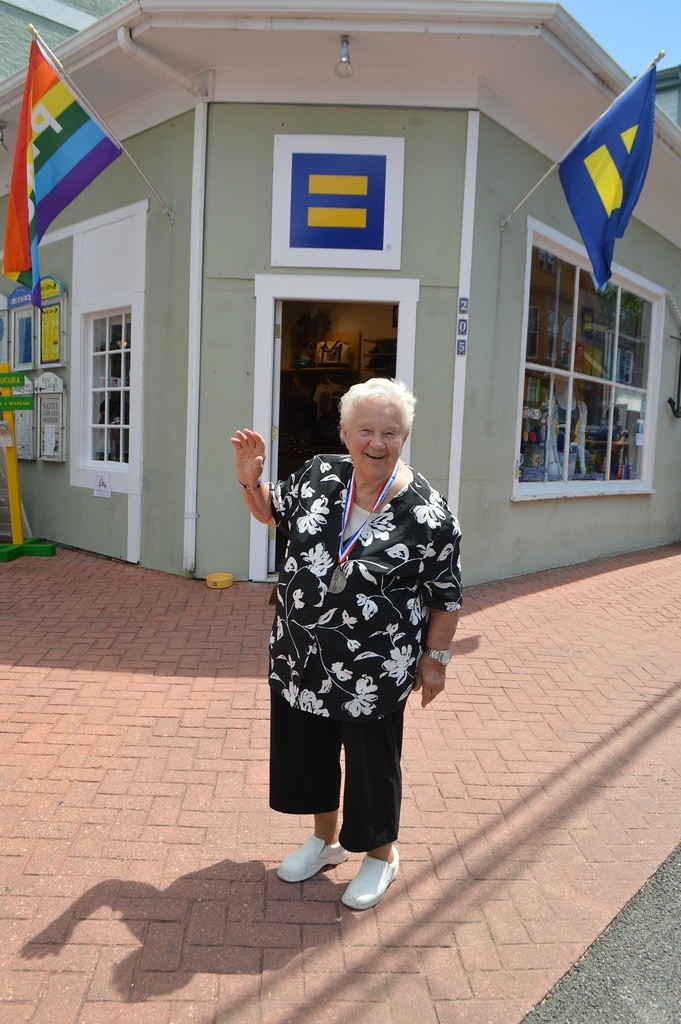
[277,836,350,882]
[342,844,399,911]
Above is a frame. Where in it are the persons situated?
[548,377,586,475]
[594,404,630,479]
[231,375,465,911]
[298,307,332,342]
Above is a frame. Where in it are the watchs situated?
[422,647,452,667]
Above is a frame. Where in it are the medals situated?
[329,572,347,594]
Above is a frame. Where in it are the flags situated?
[557,64,656,291]
[2,37,124,312]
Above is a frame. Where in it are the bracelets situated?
[238,476,263,489]
[622,432,629,439]
